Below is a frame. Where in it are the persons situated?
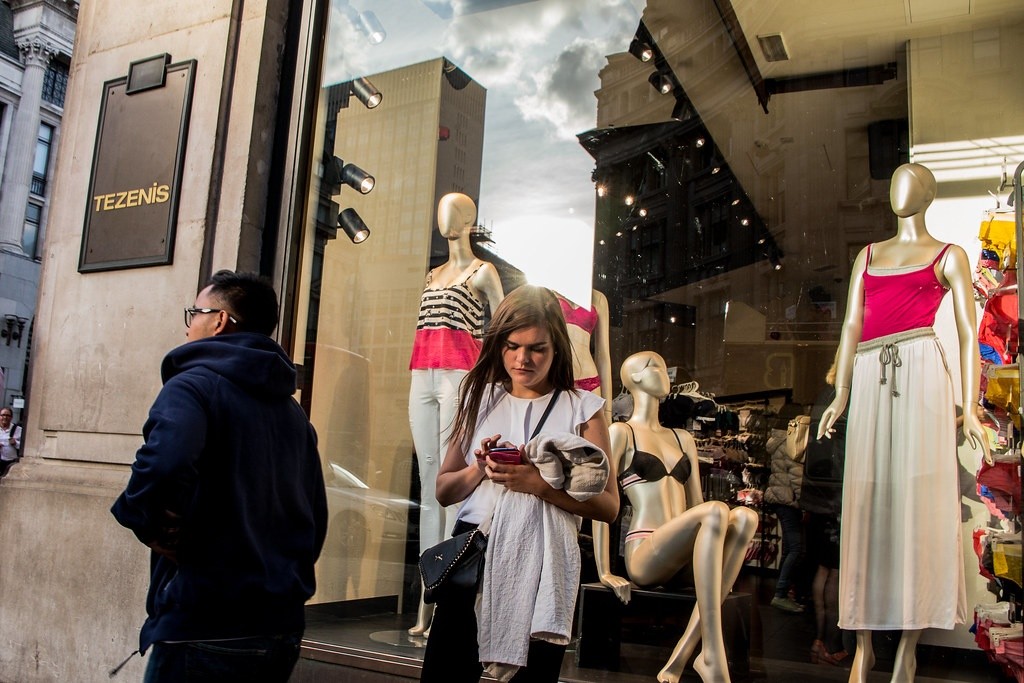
[764,391,856,667]
[524,288,632,603]
[0,407,24,477]
[591,351,762,683]
[420,285,620,683]
[111,269,329,683]
[409,193,505,638]
[815,163,994,683]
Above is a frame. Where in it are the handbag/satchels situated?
[419,529,487,603]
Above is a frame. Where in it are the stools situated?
[574,579,757,683]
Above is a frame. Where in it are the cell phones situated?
[488,448,522,484]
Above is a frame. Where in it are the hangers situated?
[616,381,774,492]
[974,186,1024,646]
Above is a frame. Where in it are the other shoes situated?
[771,596,803,612]
[811,640,825,663]
[818,644,851,667]
[790,600,807,608]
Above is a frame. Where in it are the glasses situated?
[184,307,238,327]
[1,414,12,417]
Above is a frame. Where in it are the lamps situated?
[649,71,672,93]
[707,158,725,175]
[346,74,383,109]
[755,229,769,245]
[684,129,709,148]
[741,212,756,226]
[352,11,387,46]
[728,191,743,206]
[337,163,376,194]
[672,100,689,122]
[629,40,653,62]
[771,256,784,270]
[335,209,370,243]
